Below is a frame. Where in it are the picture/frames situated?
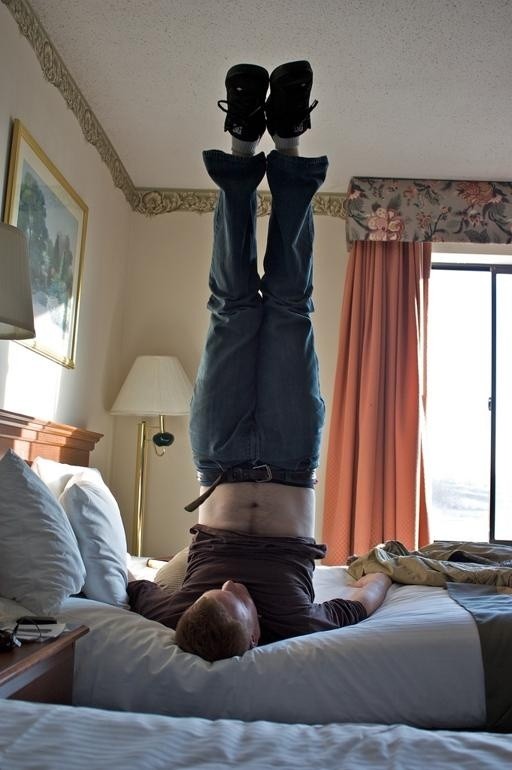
[2,119,88,370]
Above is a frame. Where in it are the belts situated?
[185,465,318,512]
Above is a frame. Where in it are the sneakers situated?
[224,64,268,143]
[266,61,314,137]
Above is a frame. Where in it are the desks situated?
[0,626,89,702]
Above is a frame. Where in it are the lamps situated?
[0,223,35,340]
[110,355,194,558]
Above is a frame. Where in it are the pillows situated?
[0,449,129,617]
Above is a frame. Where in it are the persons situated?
[121,58,394,659]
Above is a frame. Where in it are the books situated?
[7,636,50,644]
[2,620,66,637]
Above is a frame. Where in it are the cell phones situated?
[16,615,58,626]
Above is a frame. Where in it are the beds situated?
[0,696,512,770]
[0,410,512,732]
